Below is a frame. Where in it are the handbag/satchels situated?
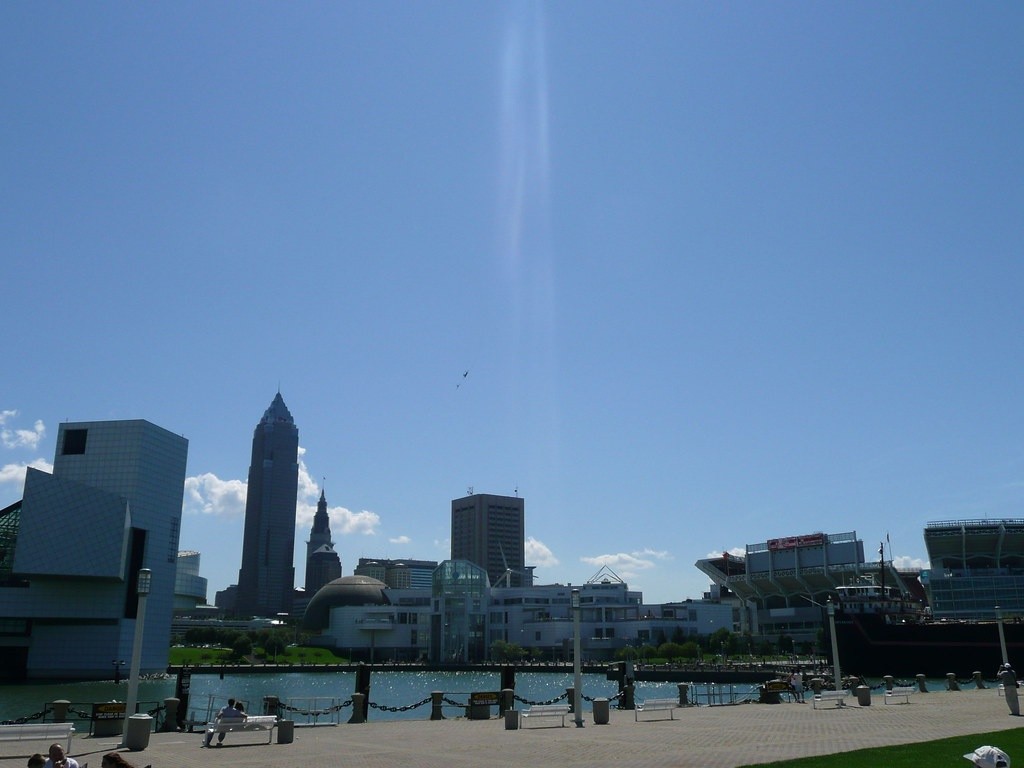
[1016,682,1019,688]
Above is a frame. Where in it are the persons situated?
[27,743,134,768]
[788,668,806,703]
[997,663,1020,716]
[201,698,247,747]
[962,745,1010,768]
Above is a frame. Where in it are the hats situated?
[962,746,1010,768]
[1004,662,1011,668]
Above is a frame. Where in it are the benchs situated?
[202,715,278,745]
[998,681,1024,696]
[0,723,75,754]
[813,690,850,709]
[519,704,571,728]
[883,687,915,705]
[634,699,680,722]
